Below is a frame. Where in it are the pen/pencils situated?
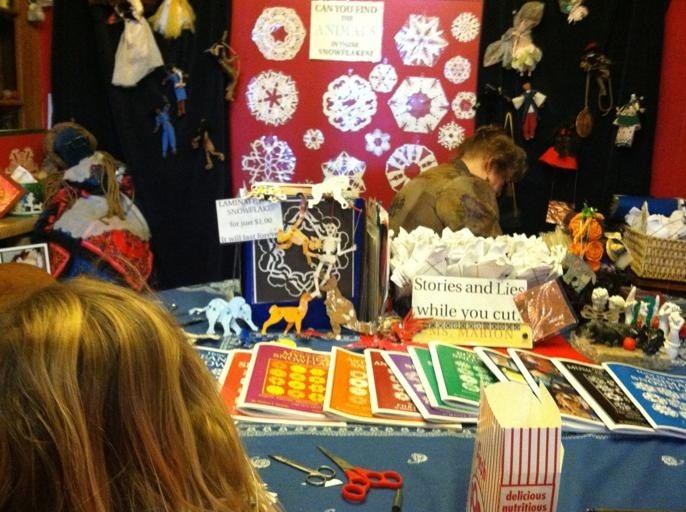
[391,489,402,512]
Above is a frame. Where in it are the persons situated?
[110,0,242,171]
[13,248,44,269]
[387,126,529,317]
[0,275,284,512]
[477,0,646,158]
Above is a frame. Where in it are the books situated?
[472,347,686,440]
[192,342,347,426]
[323,341,498,430]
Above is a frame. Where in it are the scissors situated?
[316,445,404,502]
[269,454,337,488]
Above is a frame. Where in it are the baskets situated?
[624,225,686,282]
